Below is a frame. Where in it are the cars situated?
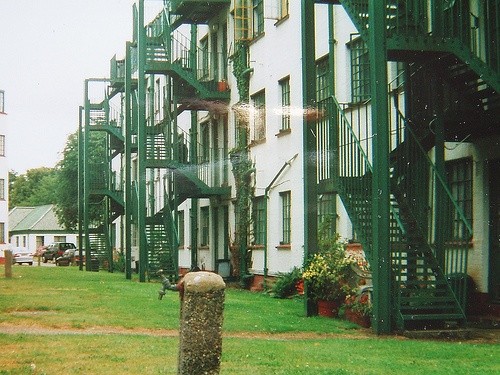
[10,247,34,265]
[55,249,97,269]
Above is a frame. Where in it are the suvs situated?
[41,242,76,264]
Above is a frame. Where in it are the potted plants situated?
[302,216,374,328]
